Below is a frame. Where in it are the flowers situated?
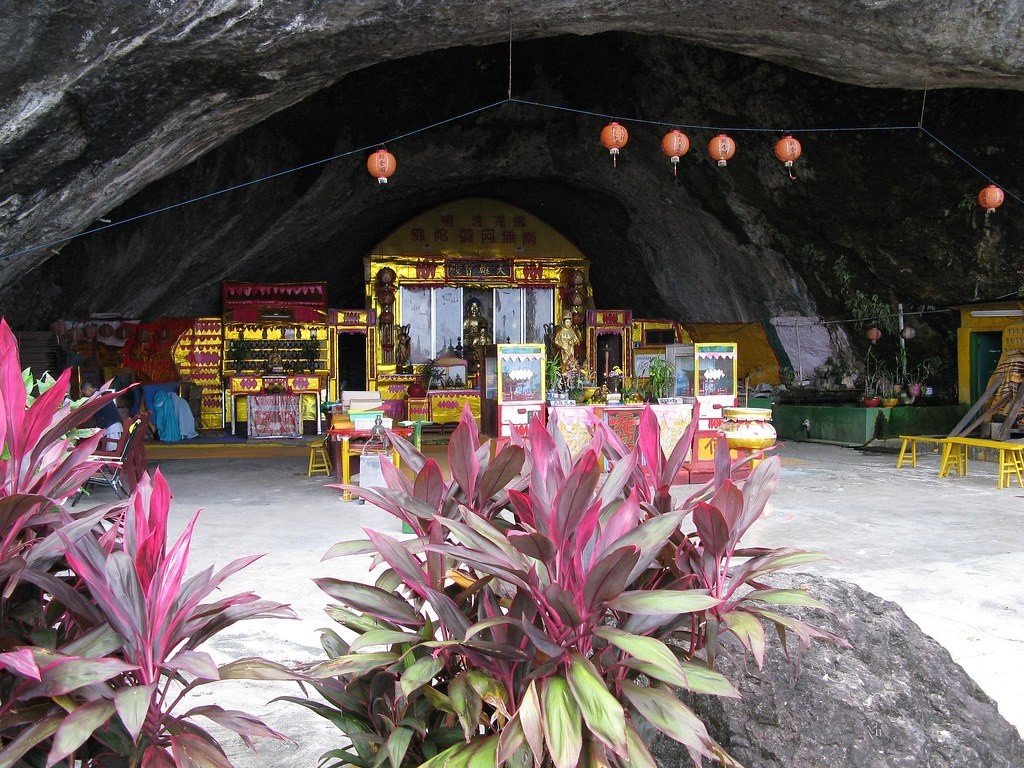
[609,366,623,387]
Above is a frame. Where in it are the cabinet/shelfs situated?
[667,342,738,418]
[484,343,546,439]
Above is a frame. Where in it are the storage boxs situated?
[403,394,428,422]
[341,391,393,430]
[359,453,393,506]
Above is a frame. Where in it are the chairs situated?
[70,411,153,507]
[188,386,203,439]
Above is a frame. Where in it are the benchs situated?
[309,439,333,476]
[896,435,1024,489]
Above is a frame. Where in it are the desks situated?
[230,376,323,435]
[547,404,694,469]
[428,389,483,422]
[246,392,304,439]
[332,413,413,501]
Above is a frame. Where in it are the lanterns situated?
[376,267,397,343]
[367,149,396,184]
[601,122,629,167]
[708,134,735,167]
[774,135,801,180]
[661,129,690,175]
[978,184,1004,213]
[566,270,586,338]
[867,326,915,344]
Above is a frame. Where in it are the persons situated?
[397,326,411,365]
[552,312,581,373]
[81,382,123,452]
[463,297,493,346]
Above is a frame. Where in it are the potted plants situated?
[420,358,446,389]
[446,374,466,390]
[859,343,932,408]
[644,352,675,397]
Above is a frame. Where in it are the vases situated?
[309,359,315,374]
[236,360,243,374]
